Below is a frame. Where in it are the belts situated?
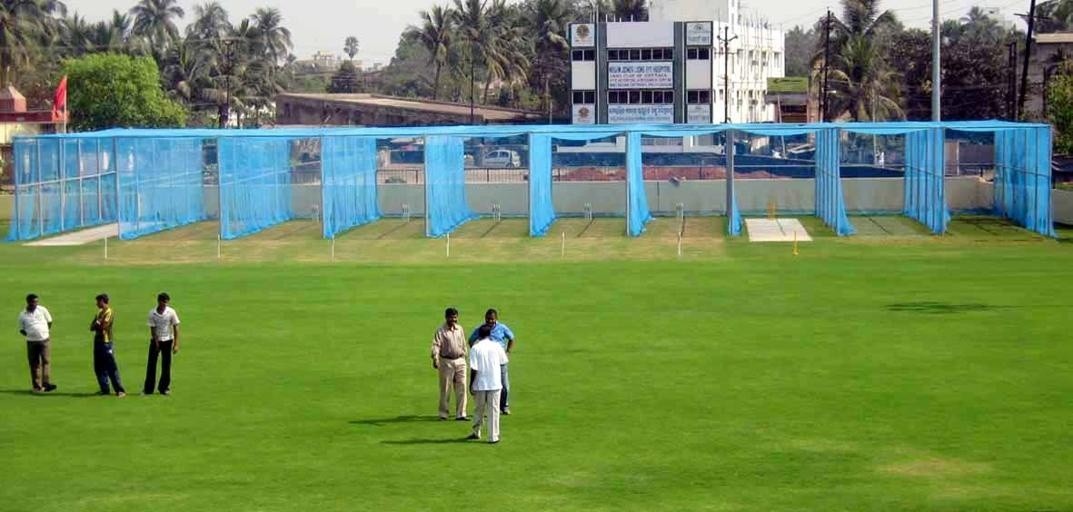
[441,355,462,360]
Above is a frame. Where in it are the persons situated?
[468,309,515,415]
[142,293,181,394]
[466,325,510,443]
[430,307,471,421]
[90,294,127,397]
[18,294,57,393]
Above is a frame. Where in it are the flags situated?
[51,76,67,123]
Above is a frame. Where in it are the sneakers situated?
[500,406,511,415]
[439,417,470,421]
[163,389,171,394]
[464,434,501,444]
[95,391,126,399]
[33,383,57,393]
